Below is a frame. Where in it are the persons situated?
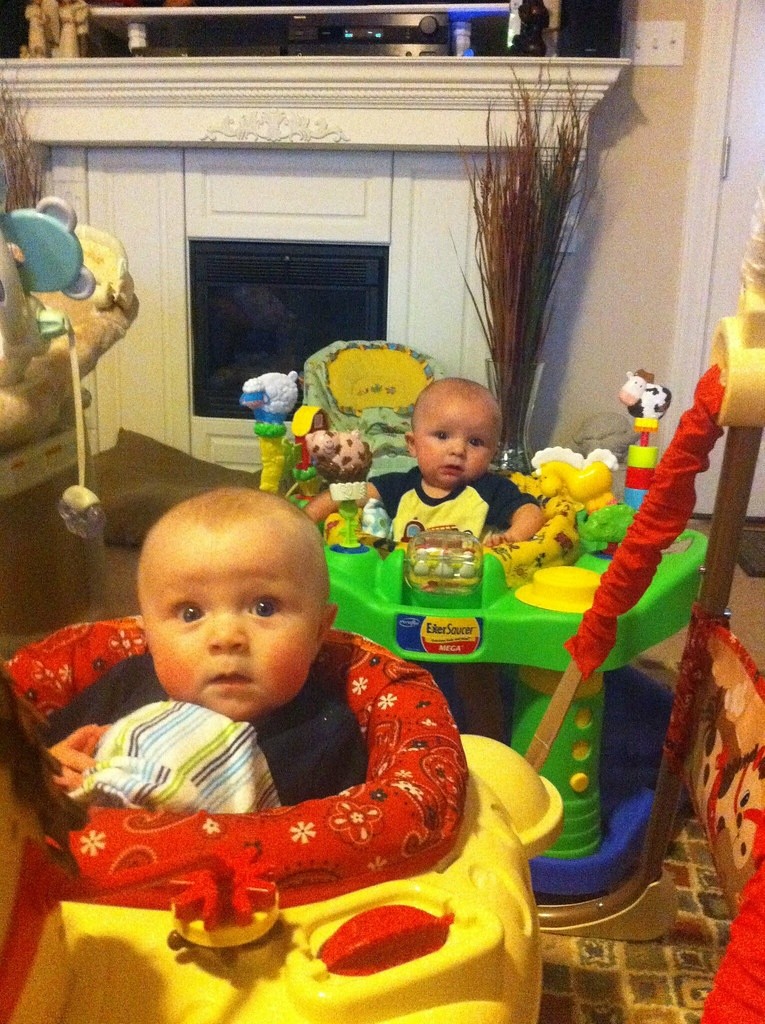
[37,486,368,806]
[302,377,549,547]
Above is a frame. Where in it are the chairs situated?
[1,615,564,1024]
[283,473,715,902]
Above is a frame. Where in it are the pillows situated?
[87,427,284,545]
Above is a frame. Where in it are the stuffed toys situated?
[306,429,372,480]
[239,371,298,494]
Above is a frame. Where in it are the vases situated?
[484,360,547,476]
